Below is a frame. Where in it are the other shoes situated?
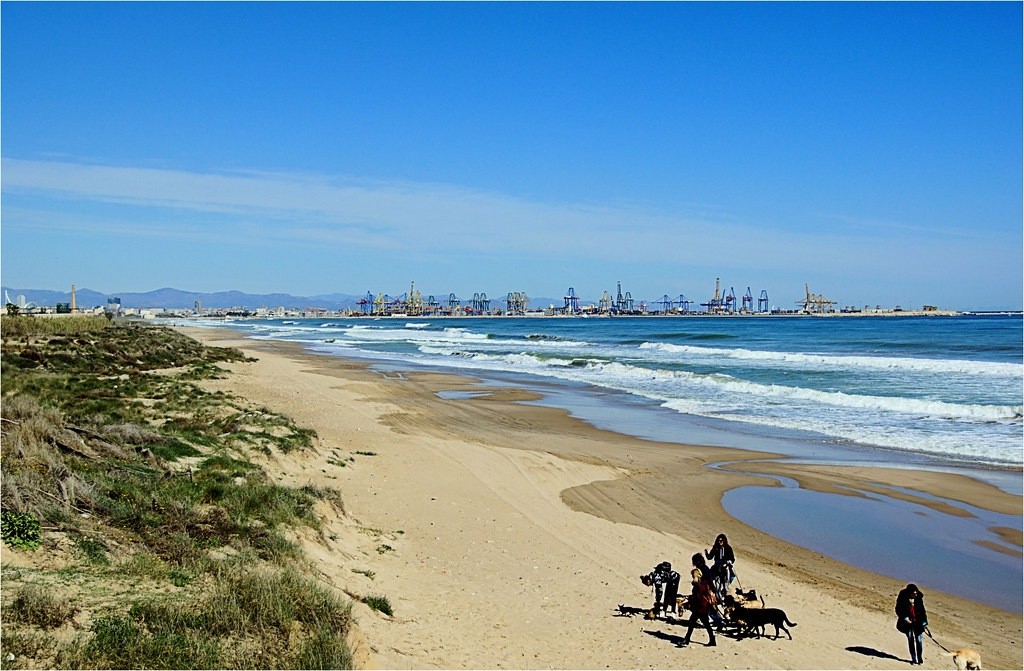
[912,658,923,666]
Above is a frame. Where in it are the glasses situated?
[718,540,723,543]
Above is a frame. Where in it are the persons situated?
[692,553,725,631]
[895,584,928,665]
[640,570,680,614]
[705,534,735,594]
[676,568,716,646]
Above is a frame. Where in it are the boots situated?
[680,636,691,645]
[703,636,716,646]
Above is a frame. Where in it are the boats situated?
[325,339,336,343]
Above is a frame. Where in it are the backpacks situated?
[707,573,725,592]
[654,561,672,573]
[701,584,718,605]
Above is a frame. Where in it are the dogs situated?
[939,648,981,671]
[664,594,688,616]
[717,588,797,640]
